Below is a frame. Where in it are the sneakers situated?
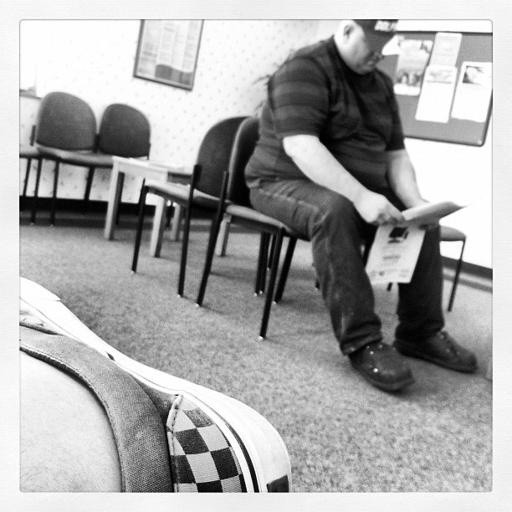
[391,328,480,374]
[21,276,293,490]
[346,341,413,391]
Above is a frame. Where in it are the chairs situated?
[20,91,465,339]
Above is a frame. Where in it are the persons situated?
[407,74,421,87]
[396,73,409,86]
[241,20,478,394]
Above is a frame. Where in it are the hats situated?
[354,20,402,57]
